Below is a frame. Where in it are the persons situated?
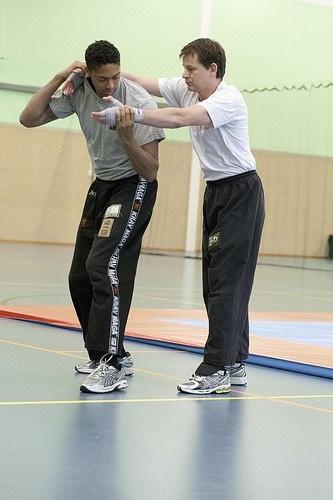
[20,41,165,393]
[61,38,265,394]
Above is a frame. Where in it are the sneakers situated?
[225,364,247,385]
[177,370,232,394]
[75,352,133,376]
[80,353,128,393]
